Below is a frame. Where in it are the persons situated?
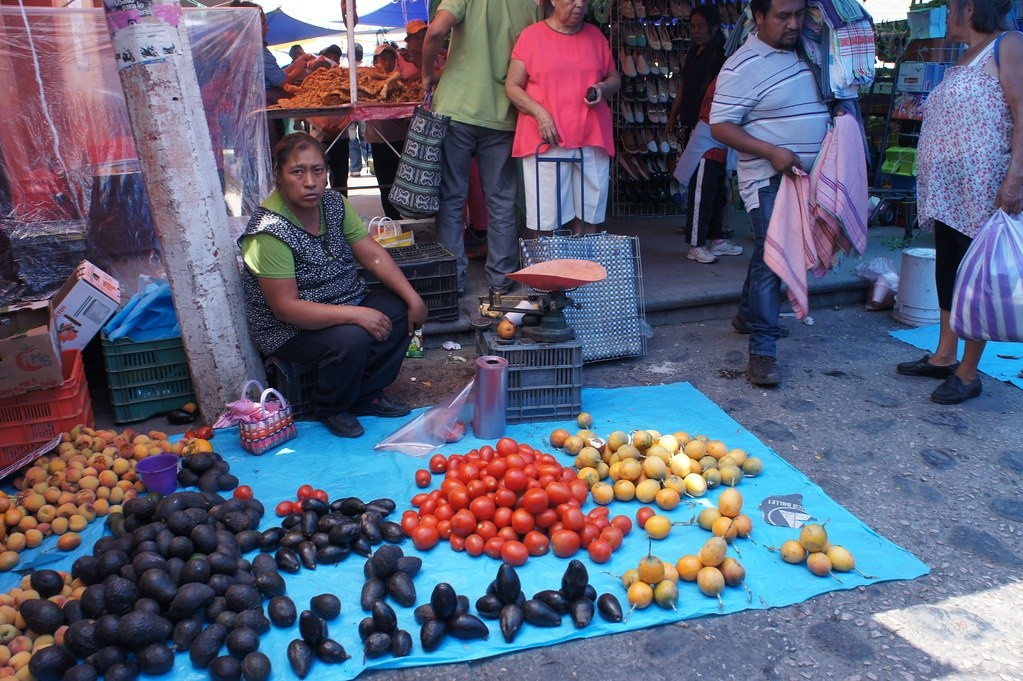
[898,1,1023,403]
[236,132,430,439]
[709,0,857,385]
[665,4,743,263]
[504,0,622,237]
[211,0,490,242]
[419,0,548,290]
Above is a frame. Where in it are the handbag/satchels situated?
[362,215,415,249]
[388,90,452,218]
[949,207,1023,342]
[238,380,297,456]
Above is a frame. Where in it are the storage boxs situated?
[905,4,949,38]
[357,241,462,321]
[860,61,953,191]
[0,141,202,468]
[475,326,583,424]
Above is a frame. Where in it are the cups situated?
[871,272,899,305]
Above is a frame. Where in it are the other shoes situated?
[582,0,740,213]
[350,171,360,177]
[368,158,376,175]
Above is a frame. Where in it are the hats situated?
[318,44,342,57]
[374,45,397,56]
[406,18,428,34]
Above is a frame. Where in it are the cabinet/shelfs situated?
[859,38,966,229]
[602,1,743,220]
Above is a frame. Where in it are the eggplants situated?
[167,409,195,424]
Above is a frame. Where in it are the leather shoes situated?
[361,391,410,418]
[897,354,960,380]
[932,374,982,405]
[316,410,363,437]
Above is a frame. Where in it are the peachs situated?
[0,571,86,681]
[1,424,192,571]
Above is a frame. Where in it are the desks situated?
[252,102,436,225]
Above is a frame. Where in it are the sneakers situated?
[687,246,719,263]
[730,313,790,338]
[750,353,780,386]
[709,239,743,256]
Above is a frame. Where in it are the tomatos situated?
[185,426,214,440]
[399,420,658,561]
[234,484,329,516]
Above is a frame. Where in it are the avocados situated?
[21,450,295,681]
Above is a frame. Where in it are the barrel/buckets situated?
[135,453,179,495]
[890,247,941,326]
